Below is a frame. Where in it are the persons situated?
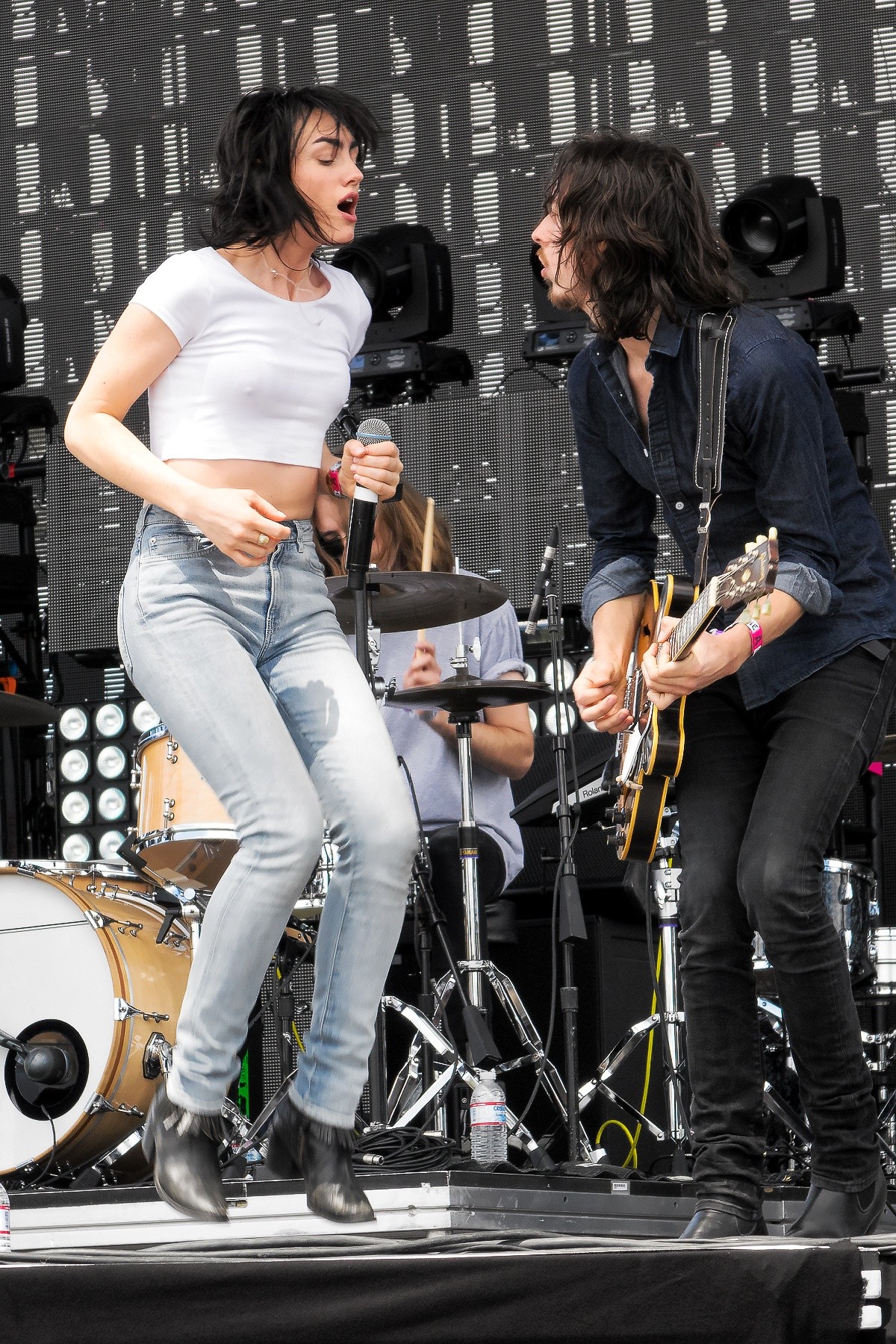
[312,448,534,975]
[65,85,417,1223]
[531,125,896,1238]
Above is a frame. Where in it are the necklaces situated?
[260,251,313,301]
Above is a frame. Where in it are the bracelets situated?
[709,618,763,661]
[326,461,349,499]
[419,710,440,721]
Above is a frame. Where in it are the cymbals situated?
[0,688,62,732]
[382,668,553,710]
[324,569,512,636]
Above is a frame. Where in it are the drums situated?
[747,857,884,999]
[0,857,201,1187]
[127,722,324,898]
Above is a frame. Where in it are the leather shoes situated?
[677,1191,768,1240]
[785,1166,888,1238]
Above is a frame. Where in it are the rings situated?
[257,533,269,545]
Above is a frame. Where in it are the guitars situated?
[604,526,780,866]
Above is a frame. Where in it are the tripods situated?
[218,697,819,1181]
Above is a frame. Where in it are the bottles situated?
[470,1067,508,1169]
[0,1184,12,1251]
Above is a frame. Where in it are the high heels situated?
[264,1096,378,1224]
[142,1083,230,1221]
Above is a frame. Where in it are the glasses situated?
[315,535,348,560]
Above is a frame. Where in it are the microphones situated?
[525,528,559,636]
[344,418,392,590]
[0,1034,76,1086]
